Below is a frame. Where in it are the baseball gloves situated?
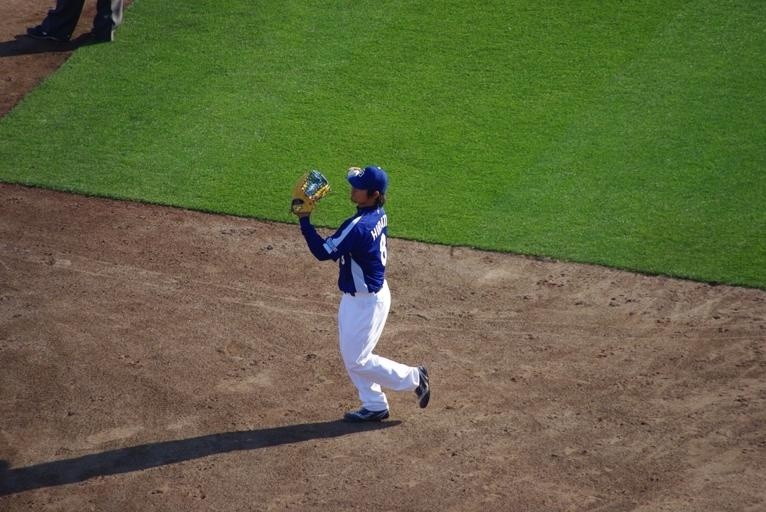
[291,169,330,214]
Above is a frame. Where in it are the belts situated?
[344,290,378,297]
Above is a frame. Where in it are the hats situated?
[346,166,388,193]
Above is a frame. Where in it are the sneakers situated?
[88,28,113,42]
[27,25,69,41]
[415,365,430,408]
[343,408,389,422]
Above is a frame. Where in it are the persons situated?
[290,163,431,424]
[26,2,125,44]
